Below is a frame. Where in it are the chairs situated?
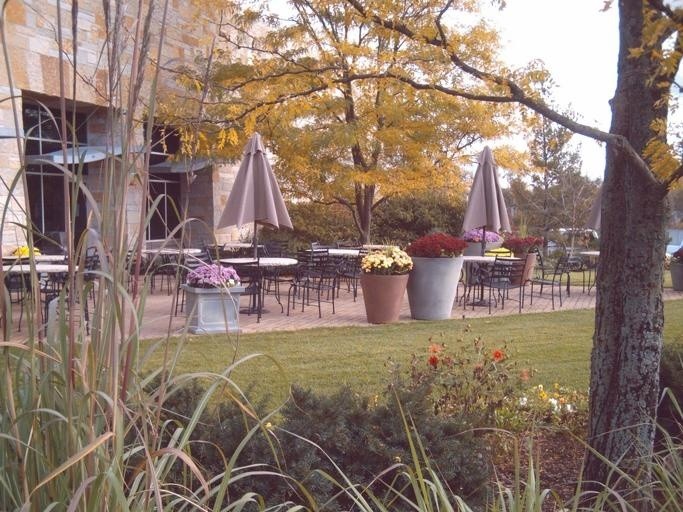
[479,255,523,314]
[294,239,361,302]
[523,249,570,311]
[560,242,585,295]
[287,258,342,317]
[40,239,224,338]
[264,243,288,294]
[218,258,264,322]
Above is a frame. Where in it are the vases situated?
[360,273,409,323]
[463,241,503,286]
[182,285,246,333]
[670,261,683,291]
[408,257,464,320]
[510,253,537,285]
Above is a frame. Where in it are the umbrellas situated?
[462,145,513,301]
[587,186,602,230]
[217,131,295,309]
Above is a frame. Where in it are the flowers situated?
[462,228,502,241]
[187,264,239,286]
[360,246,413,275]
[410,233,470,257]
[504,232,548,254]
[14,245,40,257]
[671,245,683,262]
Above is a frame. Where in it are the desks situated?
[220,244,264,283]
[220,257,297,314]
[2,255,66,262]
[1,263,78,332]
[579,251,598,295]
[463,255,520,307]
